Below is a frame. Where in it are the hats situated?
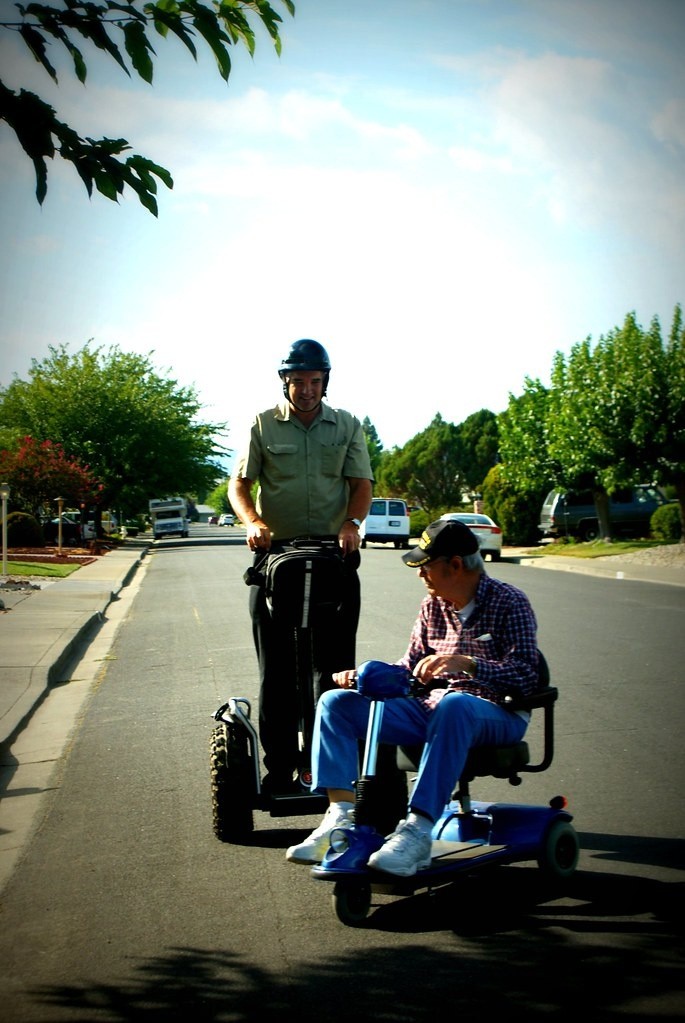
[402,519,479,568]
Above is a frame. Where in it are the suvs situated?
[537,479,680,545]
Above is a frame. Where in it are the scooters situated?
[311,642,583,928]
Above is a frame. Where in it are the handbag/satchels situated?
[266,550,348,630]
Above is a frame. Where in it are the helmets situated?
[278,339,331,382]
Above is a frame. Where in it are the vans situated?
[358,497,412,550]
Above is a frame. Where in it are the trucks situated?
[148,497,190,539]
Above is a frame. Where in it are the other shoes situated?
[262,772,293,792]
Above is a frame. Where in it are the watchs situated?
[349,518,361,526]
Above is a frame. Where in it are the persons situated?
[284,517,544,877]
[226,338,375,793]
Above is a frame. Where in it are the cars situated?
[207,514,235,527]
[31,508,119,546]
[439,512,503,562]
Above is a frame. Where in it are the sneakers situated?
[286,806,355,865]
[367,820,432,877]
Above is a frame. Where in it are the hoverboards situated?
[209,536,411,844]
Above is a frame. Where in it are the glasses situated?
[419,558,450,572]
